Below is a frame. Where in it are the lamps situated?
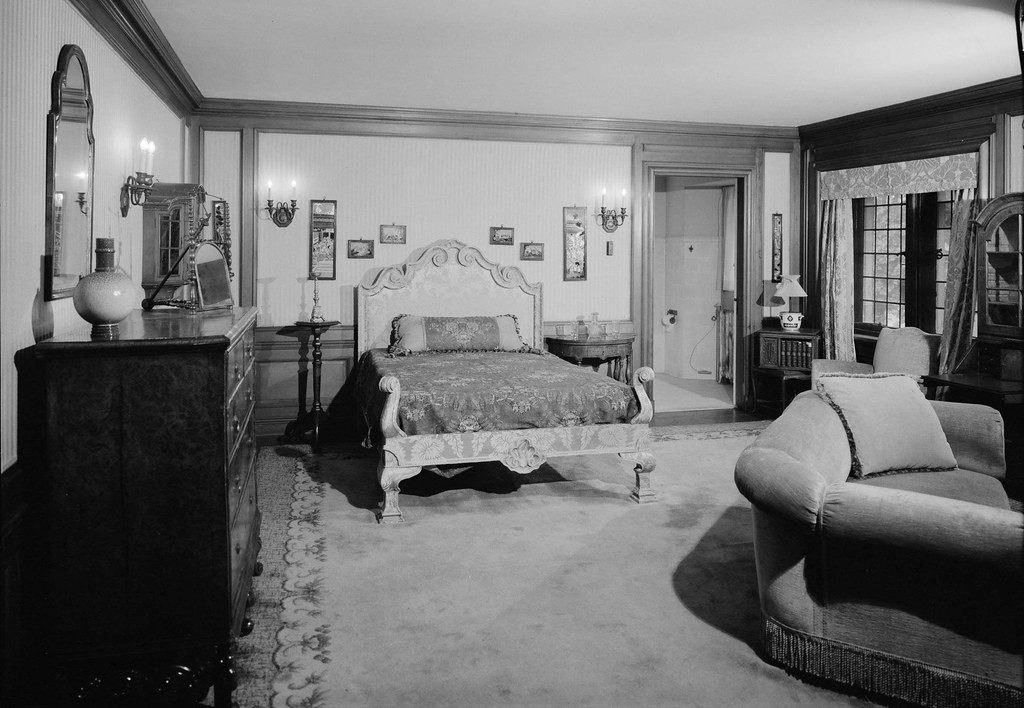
[773,274,808,331]
[597,189,629,233]
[265,179,301,228]
[120,136,157,217]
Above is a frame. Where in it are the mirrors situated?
[45,44,95,302]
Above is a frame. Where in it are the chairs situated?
[811,326,943,396]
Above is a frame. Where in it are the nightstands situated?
[546,335,635,385]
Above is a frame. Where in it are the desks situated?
[917,372,1023,464]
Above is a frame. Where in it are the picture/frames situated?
[309,199,337,280]
[380,225,407,245]
[348,240,375,259]
[520,243,545,261]
[490,227,515,246]
[563,207,588,282]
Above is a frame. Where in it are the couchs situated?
[734,391,1024,708]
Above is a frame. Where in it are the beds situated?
[353,240,659,524]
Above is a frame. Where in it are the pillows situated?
[815,370,958,479]
[390,313,533,356]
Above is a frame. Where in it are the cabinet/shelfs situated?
[16,304,263,708]
[749,328,826,414]
